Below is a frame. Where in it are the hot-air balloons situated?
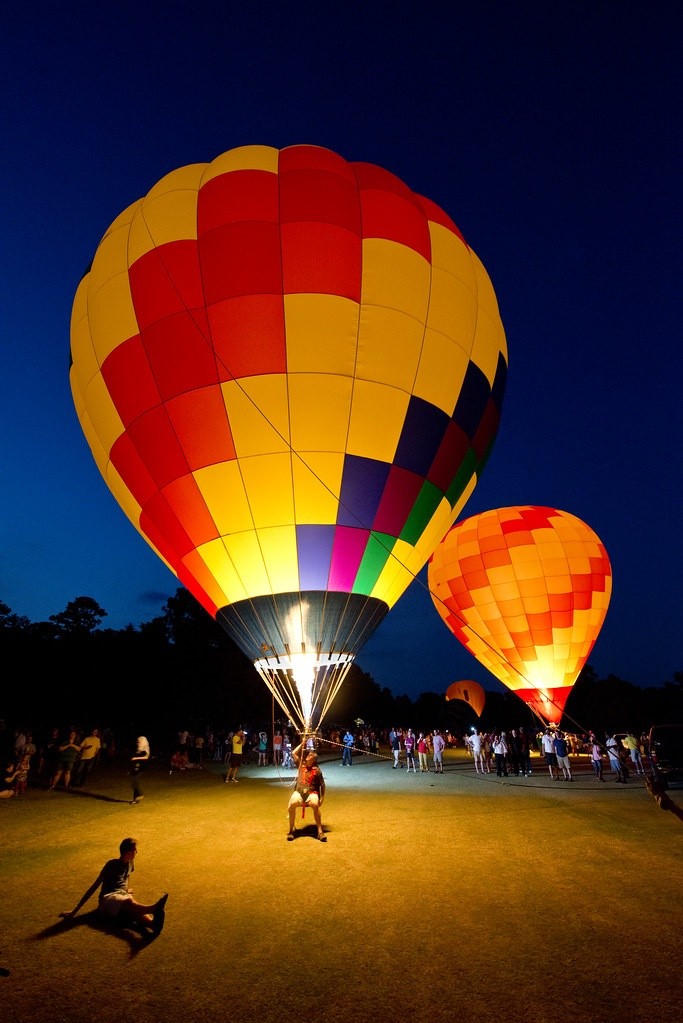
[428,504,613,733]
[445,681,486,716]
[69,145,510,738]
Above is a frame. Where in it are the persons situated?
[1,714,672,803]
[285,738,328,843]
[59,837,168,936]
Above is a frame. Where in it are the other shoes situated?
[194,766,202,770]
[594,775,606,783]
[47,786,56,791]
[150,894,169,935]
[224,778,239,784]
[129,795,144,805]
[477,767,532,778]
[316,832,327,841]
[347,764,350,767]
[615,766,662,784]
[392,761,443,774]
[340,763,343,766]
[287,828,298,841]
[550,777,574,782]
[64,786,72,792]
